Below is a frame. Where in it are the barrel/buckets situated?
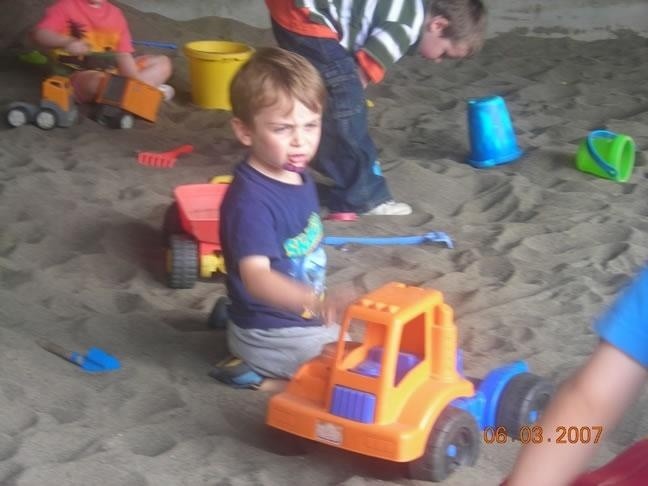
[573,130,635,183]
[466,95,526,170]
[182,39,257,111]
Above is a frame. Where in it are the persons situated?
[29,0,178,104]
[492,253,647,485]
[205,45,353,394]
[260,0,489,218]
